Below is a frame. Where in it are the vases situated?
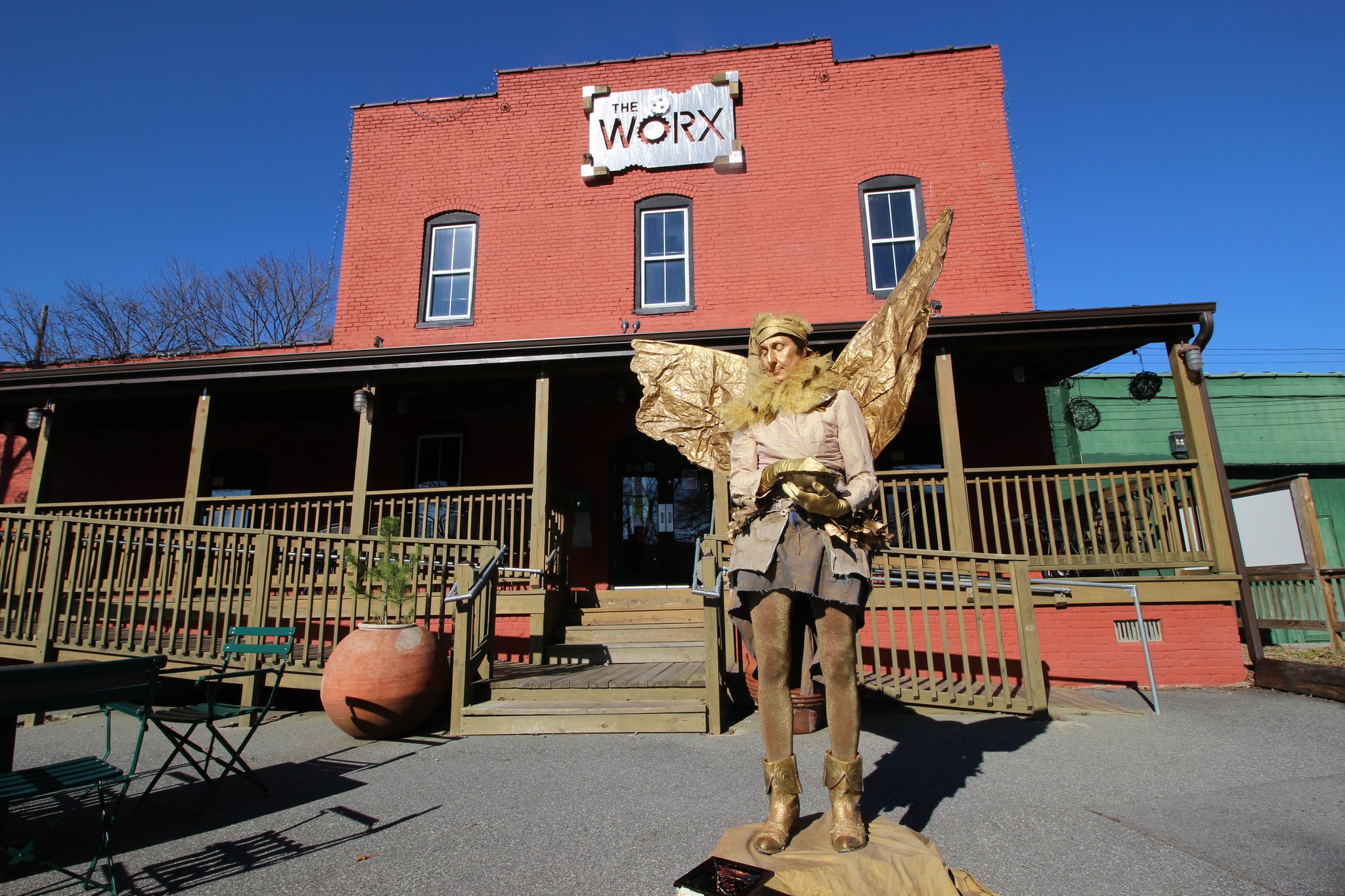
[791,695,825,735]
[744,651,801,704]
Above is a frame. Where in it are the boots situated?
[754,753,803,855]
[822,749,864,852]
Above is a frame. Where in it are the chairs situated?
[1,654,168,896]
[149,626,297,810]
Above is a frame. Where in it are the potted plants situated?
[319,516,454,738]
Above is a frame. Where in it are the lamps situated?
[373,336,384,348]
[354,389,371,414]
[1179,346,1204,372]
[632,321,640,333]
[929,300,942,310]
[621,320,629,333]
[26,407,53,428]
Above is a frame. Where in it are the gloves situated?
[758,456,832,494]
[781,481,852,518]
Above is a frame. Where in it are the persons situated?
[717,308,894,855]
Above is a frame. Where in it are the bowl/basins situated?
[777,471,845,494]
